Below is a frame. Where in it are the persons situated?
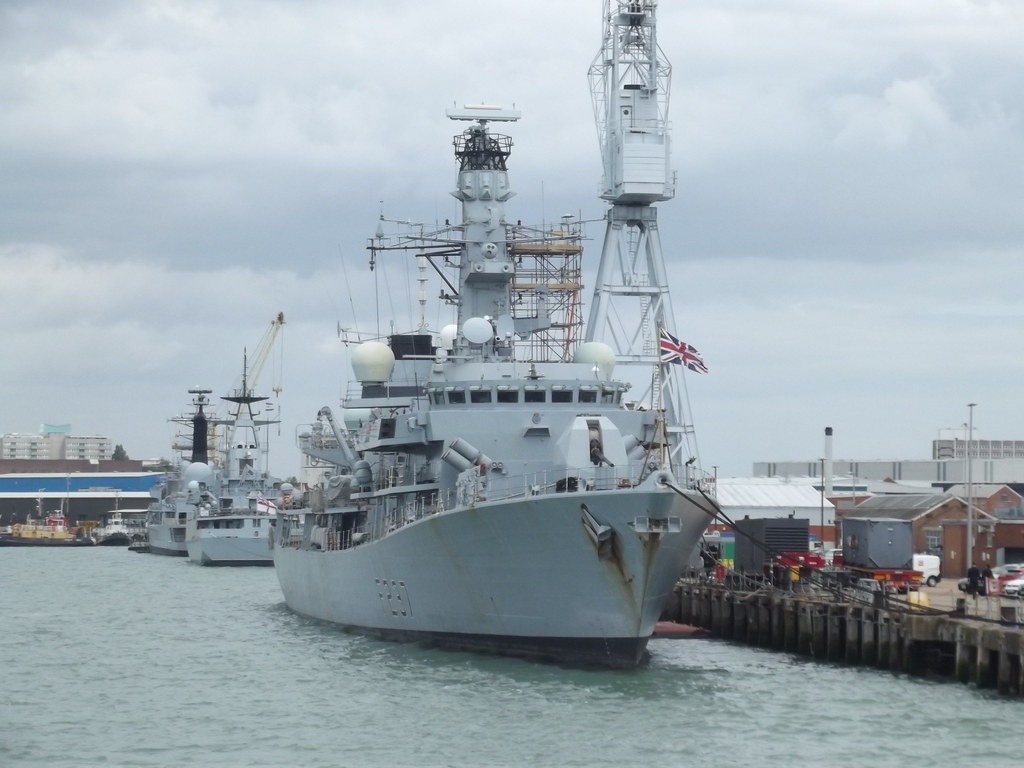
[967,561,982,600]
[982,564,994,596]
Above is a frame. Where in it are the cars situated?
[1003,574,1024,600]
[957,562,1024,595]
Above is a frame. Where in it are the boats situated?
[0,488,152,547]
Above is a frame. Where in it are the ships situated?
[270,0,726,670]
[143,308,283,569]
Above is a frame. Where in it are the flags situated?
[257,496,277,515]
[660,326,709,376]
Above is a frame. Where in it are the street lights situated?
[818,457,828,552]
[966,401,978,576]
